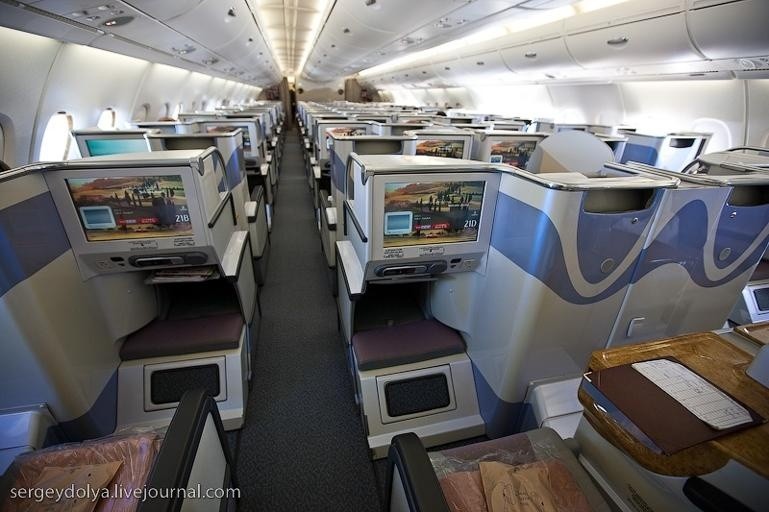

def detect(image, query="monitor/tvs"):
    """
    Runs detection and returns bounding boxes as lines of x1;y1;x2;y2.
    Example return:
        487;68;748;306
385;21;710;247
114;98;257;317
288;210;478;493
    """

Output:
64;175;194;242
206;125;251;152
490;141;536;170
416;139;464;159
86;138;148;156
325;127;366;151
384;181;486;248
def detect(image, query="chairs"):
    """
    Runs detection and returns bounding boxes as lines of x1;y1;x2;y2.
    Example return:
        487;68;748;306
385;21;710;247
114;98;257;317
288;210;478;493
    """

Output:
564;319;768;507
387;428;613;511
0;389;240;512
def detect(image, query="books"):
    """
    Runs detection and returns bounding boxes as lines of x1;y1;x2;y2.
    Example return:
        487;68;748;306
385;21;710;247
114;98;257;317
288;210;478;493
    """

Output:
581;355;768;460
